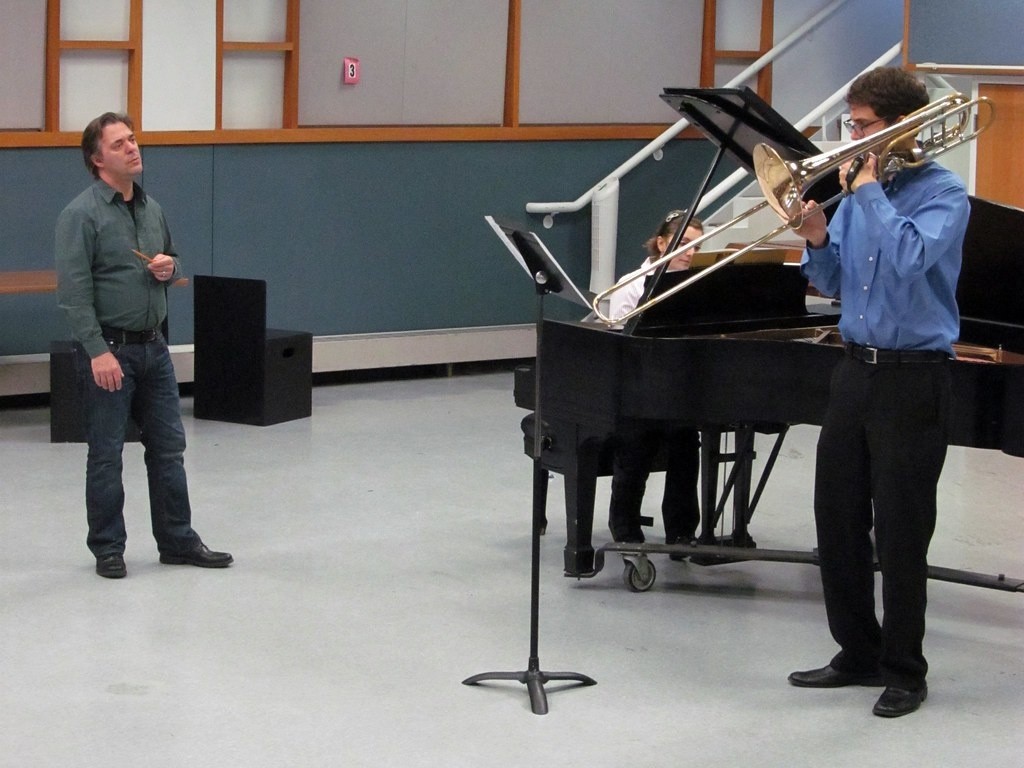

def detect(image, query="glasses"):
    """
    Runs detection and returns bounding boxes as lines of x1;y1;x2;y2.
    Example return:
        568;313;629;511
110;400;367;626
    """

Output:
844;115;889;138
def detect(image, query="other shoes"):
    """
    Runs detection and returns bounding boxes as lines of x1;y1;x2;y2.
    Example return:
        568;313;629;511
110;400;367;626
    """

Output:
607;519;646;544
663;534;699;548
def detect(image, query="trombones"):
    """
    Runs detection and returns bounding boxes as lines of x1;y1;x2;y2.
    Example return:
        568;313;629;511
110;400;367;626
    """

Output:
592;92;997;326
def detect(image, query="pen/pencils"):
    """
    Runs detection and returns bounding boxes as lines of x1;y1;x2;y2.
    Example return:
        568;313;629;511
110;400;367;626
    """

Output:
130;248;154;263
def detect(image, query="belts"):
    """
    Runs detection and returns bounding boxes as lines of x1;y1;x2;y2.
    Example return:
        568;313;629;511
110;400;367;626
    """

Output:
845;340;951;363
100;323;162;346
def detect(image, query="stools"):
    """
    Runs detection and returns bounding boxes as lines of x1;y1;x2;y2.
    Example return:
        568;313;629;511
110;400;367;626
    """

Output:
520;412;670;535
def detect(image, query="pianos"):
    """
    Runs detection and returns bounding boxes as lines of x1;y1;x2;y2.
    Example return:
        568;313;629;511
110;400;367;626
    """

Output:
512;85;1024;577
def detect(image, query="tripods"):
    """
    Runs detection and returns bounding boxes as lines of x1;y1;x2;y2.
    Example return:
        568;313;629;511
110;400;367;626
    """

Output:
461;226;597;716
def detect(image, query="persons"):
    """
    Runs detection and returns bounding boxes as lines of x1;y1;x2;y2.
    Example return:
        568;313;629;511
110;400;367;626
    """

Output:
608;209;704;561
55;111;234;579
787;67;973;715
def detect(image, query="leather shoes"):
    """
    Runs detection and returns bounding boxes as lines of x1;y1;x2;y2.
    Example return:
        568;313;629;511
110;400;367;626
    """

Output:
789;664;891;687
95;553;127;578
873;679;928;716
160;542;233;567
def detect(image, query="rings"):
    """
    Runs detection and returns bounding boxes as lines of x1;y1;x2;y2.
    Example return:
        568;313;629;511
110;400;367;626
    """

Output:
162;271;165;277
